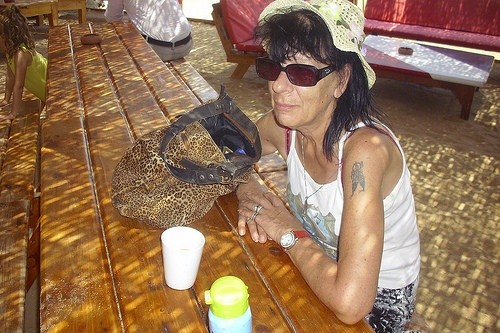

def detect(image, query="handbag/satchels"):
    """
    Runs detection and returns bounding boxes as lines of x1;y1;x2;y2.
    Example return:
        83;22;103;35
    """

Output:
111;84;262;228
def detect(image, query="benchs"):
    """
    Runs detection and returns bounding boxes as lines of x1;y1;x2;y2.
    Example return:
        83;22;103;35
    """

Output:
163;57;289;172
211;0;275;79
0;34;48;333
364;0;500;52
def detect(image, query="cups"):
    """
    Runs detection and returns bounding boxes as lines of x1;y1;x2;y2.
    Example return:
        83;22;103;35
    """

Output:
160;226;205;291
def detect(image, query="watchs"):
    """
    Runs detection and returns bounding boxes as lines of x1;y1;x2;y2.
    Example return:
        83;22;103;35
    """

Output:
280;231;311;251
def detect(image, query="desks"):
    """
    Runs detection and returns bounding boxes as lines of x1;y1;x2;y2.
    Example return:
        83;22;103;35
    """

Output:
360;33;495;120
15;0;86;26
39;19;377;333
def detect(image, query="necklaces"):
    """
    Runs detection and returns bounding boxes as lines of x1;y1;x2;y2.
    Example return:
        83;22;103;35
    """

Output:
301;134;337;214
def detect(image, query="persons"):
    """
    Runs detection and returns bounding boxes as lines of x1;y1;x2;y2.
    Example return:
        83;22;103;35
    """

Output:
105;0;193;61
235;0;420;333
0;4;48;121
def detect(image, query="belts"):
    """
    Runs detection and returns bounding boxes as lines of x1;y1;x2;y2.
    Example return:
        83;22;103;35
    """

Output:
142;33;191;47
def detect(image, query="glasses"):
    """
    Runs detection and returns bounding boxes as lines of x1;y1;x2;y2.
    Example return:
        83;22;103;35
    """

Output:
256;56;338;86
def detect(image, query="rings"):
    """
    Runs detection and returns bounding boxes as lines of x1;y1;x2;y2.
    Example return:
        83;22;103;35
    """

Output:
255;205;263;213
246;218;253;224
251;212;258;221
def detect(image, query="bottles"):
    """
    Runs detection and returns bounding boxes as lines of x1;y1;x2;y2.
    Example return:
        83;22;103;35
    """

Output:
203;276;254;333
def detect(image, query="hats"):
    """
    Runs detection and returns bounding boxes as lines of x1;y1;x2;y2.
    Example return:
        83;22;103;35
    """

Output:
258;0;376;90
